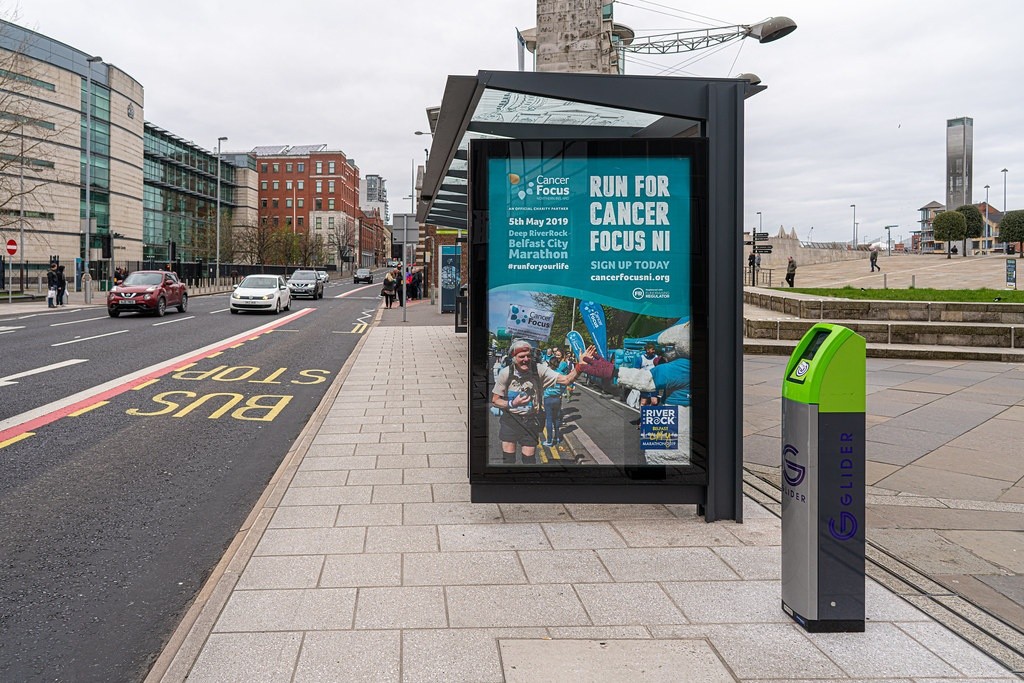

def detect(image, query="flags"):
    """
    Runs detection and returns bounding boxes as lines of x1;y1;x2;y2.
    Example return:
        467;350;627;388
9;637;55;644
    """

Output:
567;331;586;356
578;301;606;360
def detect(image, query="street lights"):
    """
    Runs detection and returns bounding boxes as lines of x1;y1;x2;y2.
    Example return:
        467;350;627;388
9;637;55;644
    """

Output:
885;227;891;256
1001;168;1008;253
984;185;990;248
17;119;29;263
217;137;228;292
864;236;867;244
855;223;859;250
84;56;102;304
850;205;855;249
757;212;762;232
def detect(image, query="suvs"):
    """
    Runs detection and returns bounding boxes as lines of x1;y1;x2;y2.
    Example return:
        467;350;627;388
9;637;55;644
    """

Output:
286;268;323;300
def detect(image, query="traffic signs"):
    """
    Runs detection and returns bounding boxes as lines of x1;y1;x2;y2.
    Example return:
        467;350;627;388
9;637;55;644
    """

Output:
755;233;769;241
744;241;755;245
755;245;773;253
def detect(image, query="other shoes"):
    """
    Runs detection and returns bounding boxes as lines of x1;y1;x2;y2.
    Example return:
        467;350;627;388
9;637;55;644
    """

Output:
389;307;392;309
49;304;57;308
384;307;388;309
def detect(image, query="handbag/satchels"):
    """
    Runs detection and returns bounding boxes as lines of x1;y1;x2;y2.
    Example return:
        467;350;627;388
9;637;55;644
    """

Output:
62;289;69;296
381;288;386;296
48;288;56;298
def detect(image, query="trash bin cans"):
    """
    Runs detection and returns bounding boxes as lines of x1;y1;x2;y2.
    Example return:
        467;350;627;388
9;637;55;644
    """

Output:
1007;244;1015;255
100;280;112;291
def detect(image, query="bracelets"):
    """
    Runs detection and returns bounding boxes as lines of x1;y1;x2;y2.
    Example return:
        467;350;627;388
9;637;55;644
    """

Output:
508;400;513;408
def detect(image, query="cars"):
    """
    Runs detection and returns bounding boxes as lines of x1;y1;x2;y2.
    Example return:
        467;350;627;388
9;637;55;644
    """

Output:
354;268;373;284
317;271;329;283
107;270;188;317
388;262;398;268
229;274;292;315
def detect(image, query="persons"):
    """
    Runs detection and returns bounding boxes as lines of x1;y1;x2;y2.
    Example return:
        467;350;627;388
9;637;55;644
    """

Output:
756;253;761;267
115;267;129;285
490;322;690;465
383;262;422;309
870;248;880;272
47;263;66;307
951;245;958;253
786;256;797;287
747;251;754;273
231;267;238;284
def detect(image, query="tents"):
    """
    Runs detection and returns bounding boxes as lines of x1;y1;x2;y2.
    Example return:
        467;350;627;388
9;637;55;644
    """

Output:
624;315;689;349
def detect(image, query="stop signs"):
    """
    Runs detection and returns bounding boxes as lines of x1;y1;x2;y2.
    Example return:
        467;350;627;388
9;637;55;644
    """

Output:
6;240;18;255
